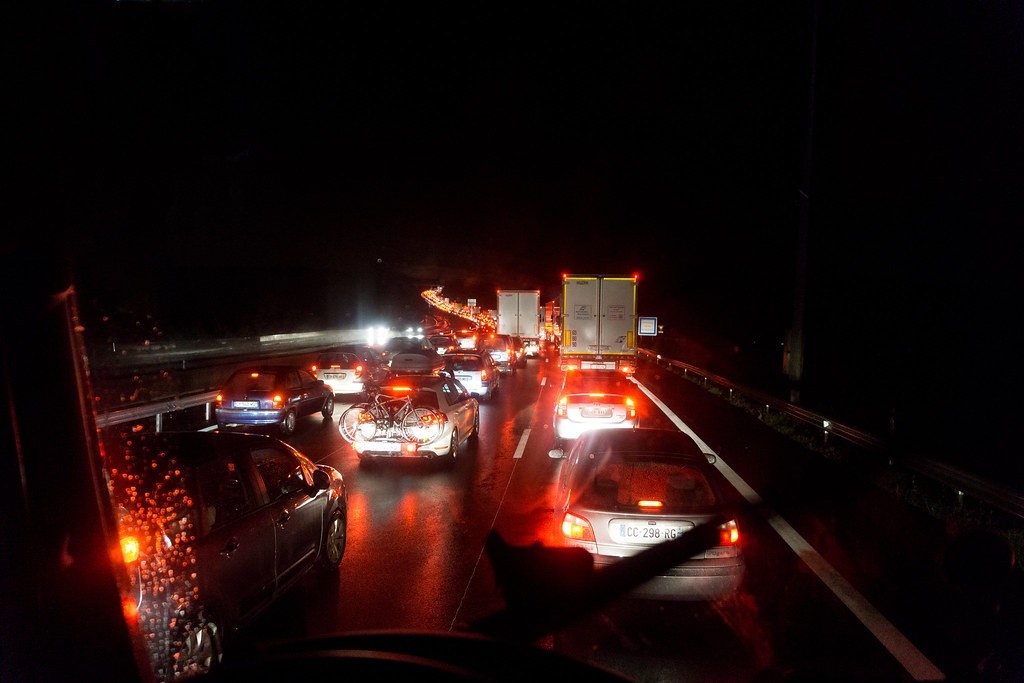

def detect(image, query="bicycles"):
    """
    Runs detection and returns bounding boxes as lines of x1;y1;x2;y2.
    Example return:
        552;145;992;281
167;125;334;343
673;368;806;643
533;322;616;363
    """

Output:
338;372;444;446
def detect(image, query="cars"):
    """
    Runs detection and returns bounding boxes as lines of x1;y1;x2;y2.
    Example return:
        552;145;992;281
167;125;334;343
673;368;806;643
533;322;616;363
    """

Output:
511;336;527;367
523;340;539;359
351;346;388;378
430;334;461;353
474;334;516;376
422;286;497;328
391;347;445;373
97;431;348;679
215;366;336;436
314;351;373;401
548;429;747;603
552;369;641;447
440;349;500;400
384;335;435;364
353;371;480;463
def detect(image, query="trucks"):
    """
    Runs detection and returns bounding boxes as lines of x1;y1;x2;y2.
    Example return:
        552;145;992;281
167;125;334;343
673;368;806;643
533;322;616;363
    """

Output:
496;288;540;339
559;274;638;376
540;301;560;349
